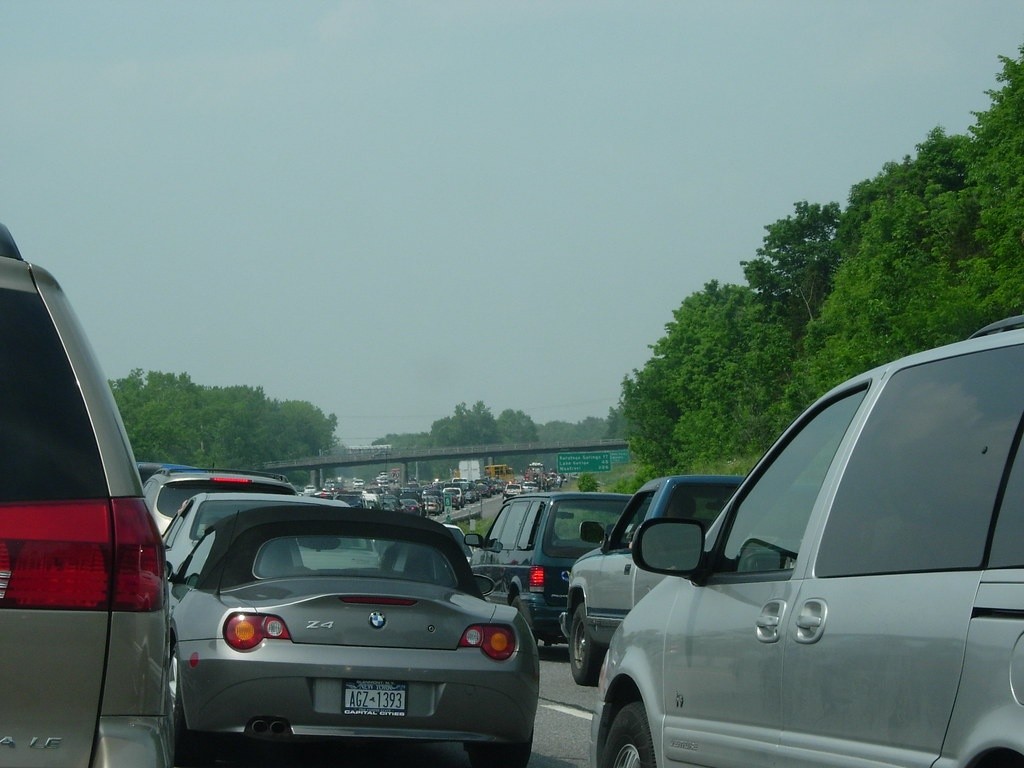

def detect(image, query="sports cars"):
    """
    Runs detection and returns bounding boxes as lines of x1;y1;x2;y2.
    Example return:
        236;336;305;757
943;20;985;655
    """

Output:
163;502;539;768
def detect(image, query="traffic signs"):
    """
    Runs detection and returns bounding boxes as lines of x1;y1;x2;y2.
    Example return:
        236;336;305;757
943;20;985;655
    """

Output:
605;449;630;464
557;452;611;475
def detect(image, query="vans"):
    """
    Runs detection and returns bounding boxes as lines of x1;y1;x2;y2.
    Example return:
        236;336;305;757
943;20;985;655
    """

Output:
464;488;633;659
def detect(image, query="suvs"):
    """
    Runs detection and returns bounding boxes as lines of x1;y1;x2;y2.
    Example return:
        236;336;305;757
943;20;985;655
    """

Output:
138;467;300;561
559;474;757;687
585;317;1024;766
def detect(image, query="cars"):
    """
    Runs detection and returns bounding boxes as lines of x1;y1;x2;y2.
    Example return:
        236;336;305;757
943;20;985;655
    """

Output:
131;461;195;498
1;220;183;766
301;451;565;517
440;523;477;570
163;493;357;620
